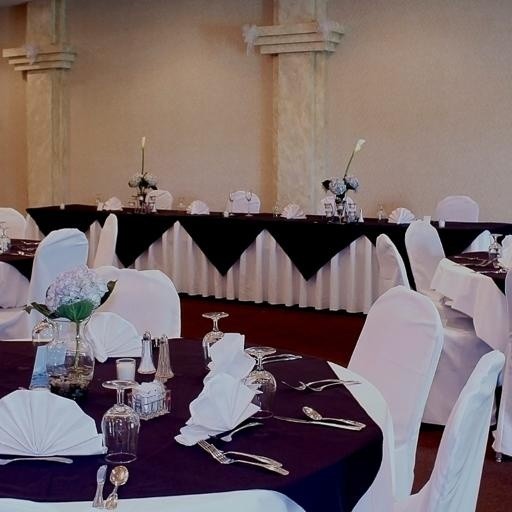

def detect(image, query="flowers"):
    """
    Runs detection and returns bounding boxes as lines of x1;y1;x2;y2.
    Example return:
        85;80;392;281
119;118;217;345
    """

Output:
127;135;159;191
23;263;120;324
318;136;370;199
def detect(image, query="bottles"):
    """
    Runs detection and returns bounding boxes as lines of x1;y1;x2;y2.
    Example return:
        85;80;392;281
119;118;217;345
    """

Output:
155;335;174;379
137;329;157;374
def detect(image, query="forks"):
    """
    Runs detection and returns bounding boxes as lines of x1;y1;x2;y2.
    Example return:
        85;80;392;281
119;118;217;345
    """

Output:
460;260;492;267
206;444;290;476
281;379;342;391
208;422;263;443
197;439;283;467
299;380;361;392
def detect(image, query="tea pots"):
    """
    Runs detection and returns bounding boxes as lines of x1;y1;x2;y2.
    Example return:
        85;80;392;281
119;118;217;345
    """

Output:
30;315;95;401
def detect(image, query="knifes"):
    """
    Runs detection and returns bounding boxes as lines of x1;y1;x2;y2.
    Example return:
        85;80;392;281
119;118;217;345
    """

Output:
256;352;301;366
91;464;109;507
274;415;362;431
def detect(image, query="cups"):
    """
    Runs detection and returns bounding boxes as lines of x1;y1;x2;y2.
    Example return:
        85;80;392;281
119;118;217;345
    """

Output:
116;358;137;395
438;216;446;228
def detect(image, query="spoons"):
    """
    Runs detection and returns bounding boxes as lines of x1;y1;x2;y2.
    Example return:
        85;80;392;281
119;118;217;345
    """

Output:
303;406;367;428
0;456;74;466
106;465;128;510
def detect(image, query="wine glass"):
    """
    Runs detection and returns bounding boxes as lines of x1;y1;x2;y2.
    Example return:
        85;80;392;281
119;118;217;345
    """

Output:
489;233;502;259
101;380;141;464
201;312;228;369
60;189;384;222
245;346;276;419
0;221;12;254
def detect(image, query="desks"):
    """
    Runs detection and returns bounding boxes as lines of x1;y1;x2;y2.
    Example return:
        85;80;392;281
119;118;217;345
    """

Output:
28;201;511;318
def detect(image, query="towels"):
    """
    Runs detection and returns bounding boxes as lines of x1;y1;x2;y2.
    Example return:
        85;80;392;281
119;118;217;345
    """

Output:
1;386;114;458
386;206;414;226
85;309;145;365
280;202;307;221
172;368;268;446
207;331;255;377
186;198;209;216
101;197;123;211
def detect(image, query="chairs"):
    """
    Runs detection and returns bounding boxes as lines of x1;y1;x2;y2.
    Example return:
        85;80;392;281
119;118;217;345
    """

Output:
145;190;176;212
389;348;506;510
427;193;482;223
225;190;261;214
375;220;510;465
345;284;447;495
68;266;185;343
0;206;128;309
0;227;91;341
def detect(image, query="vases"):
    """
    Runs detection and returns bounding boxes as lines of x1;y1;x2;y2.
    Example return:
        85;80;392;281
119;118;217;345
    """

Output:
135;193;148;213
43;319;95;394
334;201;346;222
